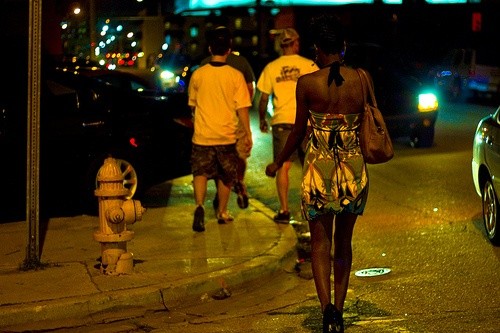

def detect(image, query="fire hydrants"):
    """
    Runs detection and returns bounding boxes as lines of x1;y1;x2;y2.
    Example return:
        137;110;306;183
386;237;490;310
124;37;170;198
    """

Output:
94;158;147;276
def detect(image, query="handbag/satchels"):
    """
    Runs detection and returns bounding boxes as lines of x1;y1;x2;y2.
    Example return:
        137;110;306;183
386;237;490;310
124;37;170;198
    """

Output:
355;68;394;164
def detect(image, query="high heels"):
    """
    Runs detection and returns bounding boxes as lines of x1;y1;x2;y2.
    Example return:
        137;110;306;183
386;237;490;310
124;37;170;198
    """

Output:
337;312;344;332
323;303;339;332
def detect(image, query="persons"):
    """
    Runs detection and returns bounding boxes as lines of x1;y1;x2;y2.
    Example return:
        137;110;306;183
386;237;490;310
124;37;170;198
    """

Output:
187;31;252;229
257;28;320;224
200;24;255;213
264;20;377;332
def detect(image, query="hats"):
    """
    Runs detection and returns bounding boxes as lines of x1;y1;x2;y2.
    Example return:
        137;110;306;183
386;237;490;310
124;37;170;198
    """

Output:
279;28;300;45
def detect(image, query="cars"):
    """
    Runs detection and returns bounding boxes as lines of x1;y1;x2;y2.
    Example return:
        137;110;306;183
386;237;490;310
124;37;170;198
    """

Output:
365;78;438;148
171;54;190;76
1;66;192;205
472;107;500;245
231;50;263;71
429;49;500;102
49;58;153;86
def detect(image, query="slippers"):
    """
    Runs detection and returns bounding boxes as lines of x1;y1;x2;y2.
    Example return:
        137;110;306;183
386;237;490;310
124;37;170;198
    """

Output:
192;204;205;232
216;211;234;224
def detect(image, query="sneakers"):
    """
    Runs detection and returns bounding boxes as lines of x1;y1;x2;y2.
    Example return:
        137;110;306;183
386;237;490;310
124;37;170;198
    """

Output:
274;209;290;223
233;180;249;209
